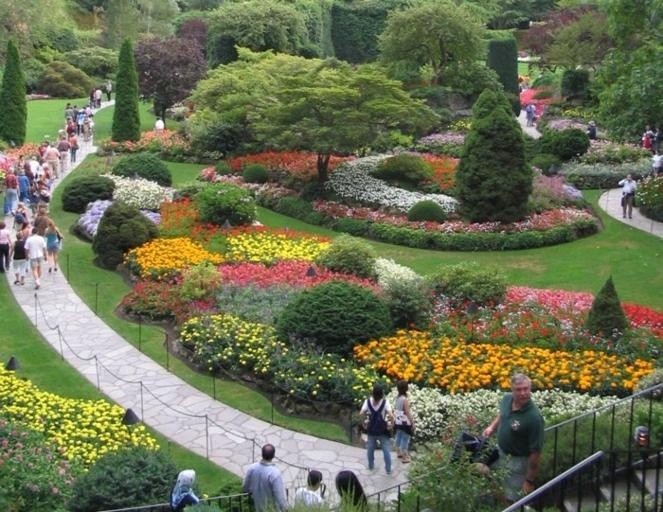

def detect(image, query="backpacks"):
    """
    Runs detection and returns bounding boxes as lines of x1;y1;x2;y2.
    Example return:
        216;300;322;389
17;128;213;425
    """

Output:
367;398;386;435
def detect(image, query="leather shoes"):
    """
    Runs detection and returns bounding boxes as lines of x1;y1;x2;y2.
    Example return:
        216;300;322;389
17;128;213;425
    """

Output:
13;267;58;289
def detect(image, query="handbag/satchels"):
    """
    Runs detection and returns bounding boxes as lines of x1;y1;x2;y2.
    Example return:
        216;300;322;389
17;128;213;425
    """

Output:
393;409;411;426
73;144;79;149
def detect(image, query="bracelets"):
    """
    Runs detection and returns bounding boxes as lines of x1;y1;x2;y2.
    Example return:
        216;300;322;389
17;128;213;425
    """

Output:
525;478;535;485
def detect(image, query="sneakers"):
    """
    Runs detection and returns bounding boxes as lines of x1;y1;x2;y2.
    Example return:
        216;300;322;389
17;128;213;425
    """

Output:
397;455;411;463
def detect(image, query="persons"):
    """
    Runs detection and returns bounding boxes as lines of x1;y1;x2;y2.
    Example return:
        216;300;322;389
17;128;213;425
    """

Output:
0;87;101;291
105;80;112;102
525;103;533;127
392;378;416;463
357;383;395;475
480;372;545;502
154;117;166;135
642;124;662;177
528;102;537;127
170;468;200;511
289;469;325;506
241;443;291;512
617;174;637;219
586;120;596;139
538;71;545;78
523;74;529;89
331;470;370;511
